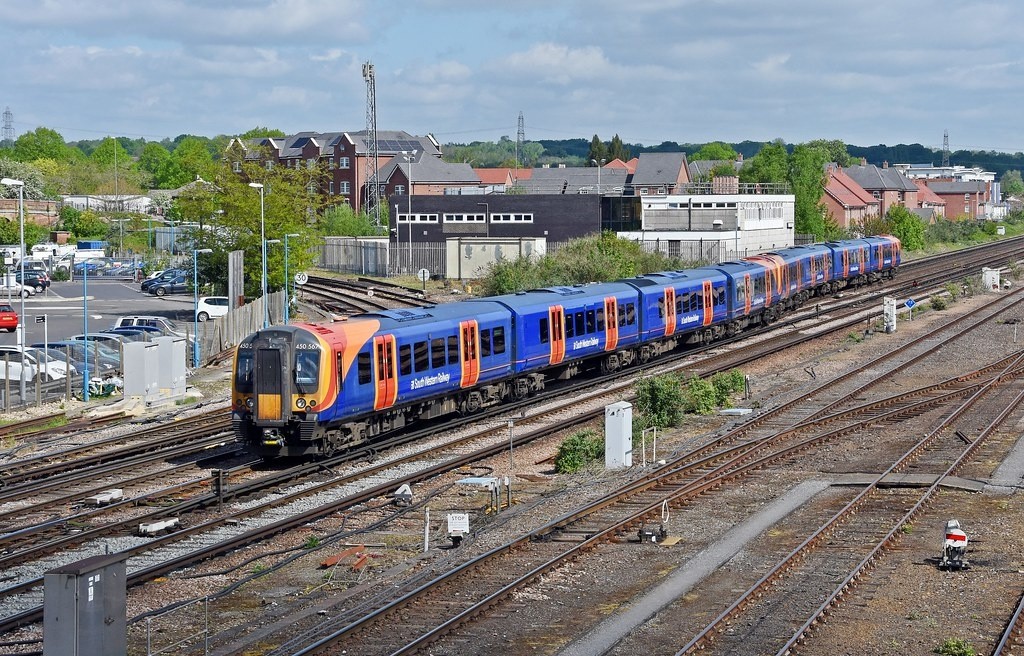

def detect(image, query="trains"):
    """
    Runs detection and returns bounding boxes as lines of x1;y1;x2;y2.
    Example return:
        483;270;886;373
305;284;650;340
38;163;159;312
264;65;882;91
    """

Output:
229;233;903;462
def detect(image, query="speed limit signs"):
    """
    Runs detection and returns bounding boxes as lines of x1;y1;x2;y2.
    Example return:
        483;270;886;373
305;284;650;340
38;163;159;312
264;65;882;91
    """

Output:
293;272;308;285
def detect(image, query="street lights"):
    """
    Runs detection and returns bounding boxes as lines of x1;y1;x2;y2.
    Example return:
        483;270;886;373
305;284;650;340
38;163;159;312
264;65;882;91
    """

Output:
477;202;489;237
262;240;281;328
0;176;27;408
400;149;419;276
283;234;300;325
248;181;266;295
111;136;118;212
73;261;90;402
590;159;607;195
193;248;213;369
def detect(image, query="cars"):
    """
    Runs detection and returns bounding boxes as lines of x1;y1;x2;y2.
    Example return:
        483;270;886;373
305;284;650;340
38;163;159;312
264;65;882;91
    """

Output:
197;296;230;322
0;315;200;395
12;248;210;296
0;303;19;332
0;277;37;299
